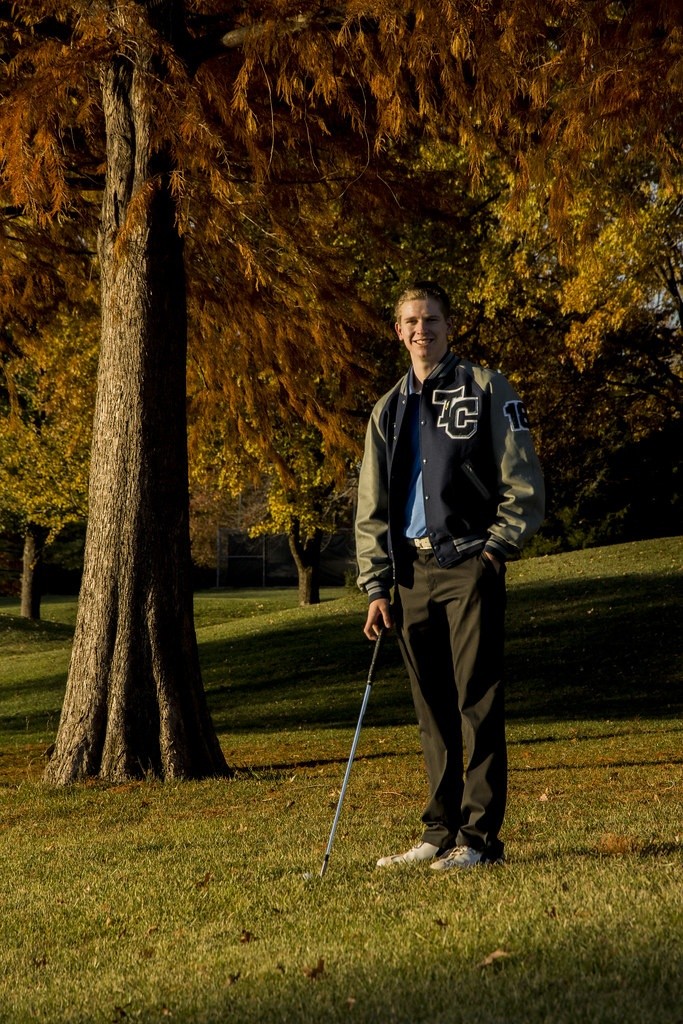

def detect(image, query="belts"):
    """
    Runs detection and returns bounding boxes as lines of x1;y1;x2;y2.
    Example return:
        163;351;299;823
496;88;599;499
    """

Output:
406;536;432;550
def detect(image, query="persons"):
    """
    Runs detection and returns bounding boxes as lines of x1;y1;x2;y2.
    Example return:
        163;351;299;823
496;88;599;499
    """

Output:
356;279;543;872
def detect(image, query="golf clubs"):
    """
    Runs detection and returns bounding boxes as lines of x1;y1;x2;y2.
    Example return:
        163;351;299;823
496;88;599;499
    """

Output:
301;624;387;885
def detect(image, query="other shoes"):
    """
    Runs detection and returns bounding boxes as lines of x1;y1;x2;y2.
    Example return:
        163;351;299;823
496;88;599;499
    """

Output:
429;845;506;870
376;840;457;869
